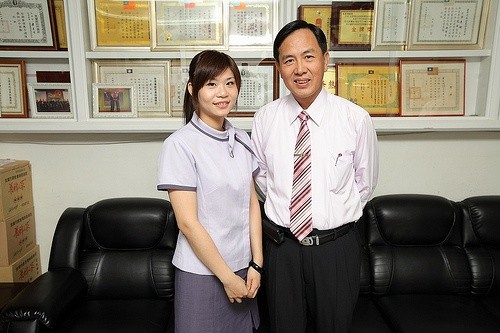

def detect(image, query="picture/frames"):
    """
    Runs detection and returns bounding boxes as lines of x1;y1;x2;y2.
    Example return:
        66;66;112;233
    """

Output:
26;83;73;119
92;83;137;118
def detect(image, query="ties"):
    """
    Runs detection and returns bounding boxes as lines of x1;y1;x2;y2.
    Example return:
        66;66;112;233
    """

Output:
290;111;313;241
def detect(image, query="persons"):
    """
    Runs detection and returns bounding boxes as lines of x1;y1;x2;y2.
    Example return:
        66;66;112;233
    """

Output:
35;98;70;112
252;19;379;333
152;47;264;333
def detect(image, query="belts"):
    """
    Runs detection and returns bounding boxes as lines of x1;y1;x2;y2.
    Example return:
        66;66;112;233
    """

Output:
283;222;355;246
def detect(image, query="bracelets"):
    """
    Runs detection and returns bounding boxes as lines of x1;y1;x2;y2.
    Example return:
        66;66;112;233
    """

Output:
249;261;263;274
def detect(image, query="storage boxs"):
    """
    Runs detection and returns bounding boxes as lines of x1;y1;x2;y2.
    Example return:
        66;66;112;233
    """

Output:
0;159;41;283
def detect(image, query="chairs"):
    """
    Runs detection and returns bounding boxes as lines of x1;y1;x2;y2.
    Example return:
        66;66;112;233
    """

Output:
4;198;177;333
356;193;500;333
